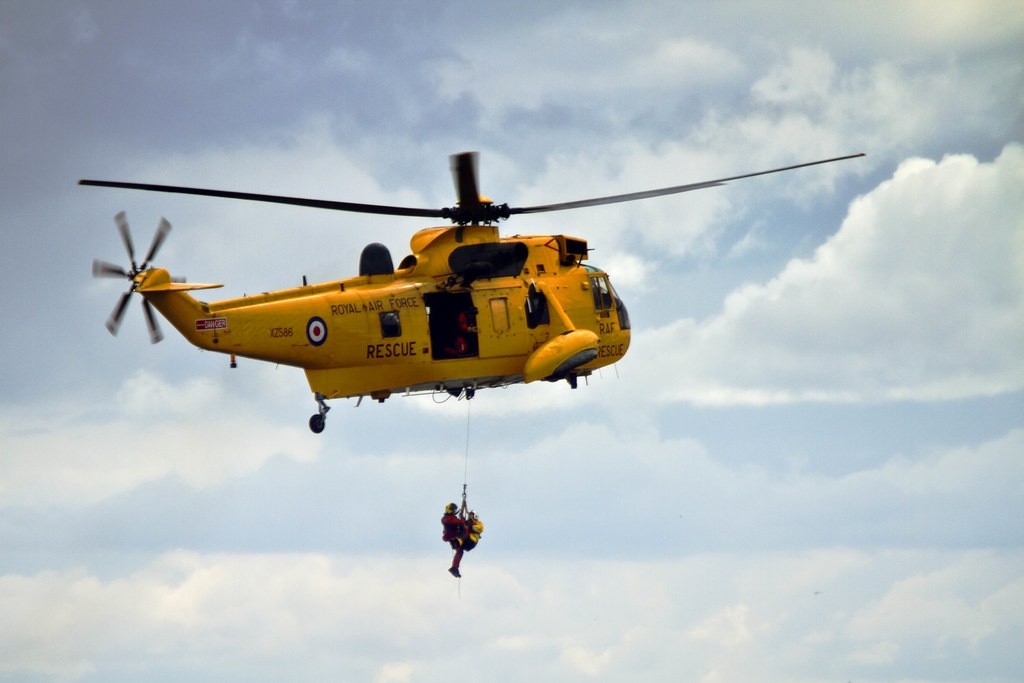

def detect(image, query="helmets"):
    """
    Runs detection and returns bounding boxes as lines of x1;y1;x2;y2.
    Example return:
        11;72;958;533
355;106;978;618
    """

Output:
444;503;458;514
468;510;479;520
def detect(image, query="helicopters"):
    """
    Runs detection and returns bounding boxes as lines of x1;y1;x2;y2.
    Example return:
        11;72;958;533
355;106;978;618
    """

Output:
76;148;865;435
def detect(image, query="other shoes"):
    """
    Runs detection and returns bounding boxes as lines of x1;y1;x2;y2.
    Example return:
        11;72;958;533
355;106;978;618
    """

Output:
448;567;461;578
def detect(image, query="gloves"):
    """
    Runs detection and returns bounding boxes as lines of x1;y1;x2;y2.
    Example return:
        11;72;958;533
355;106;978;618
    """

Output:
472;326;480;333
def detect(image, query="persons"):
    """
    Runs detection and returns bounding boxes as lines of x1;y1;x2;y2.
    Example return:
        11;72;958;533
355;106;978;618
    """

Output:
441;503;484;577
443;304;478;354
